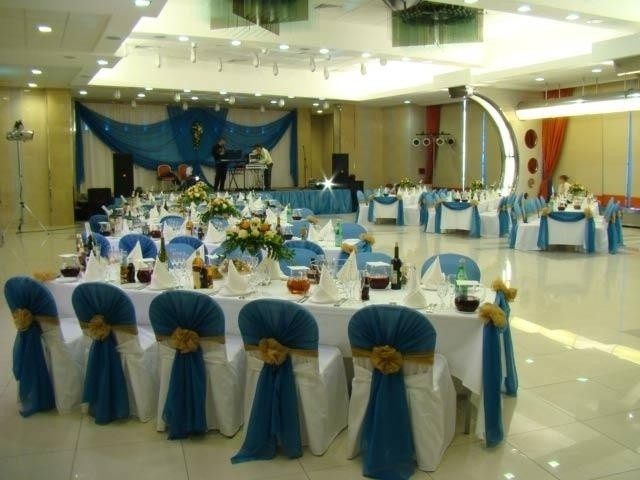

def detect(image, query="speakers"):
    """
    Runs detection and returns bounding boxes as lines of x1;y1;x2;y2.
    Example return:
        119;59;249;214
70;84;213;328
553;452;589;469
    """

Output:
113;152;134;198
88;187;111;201
332;153;348;182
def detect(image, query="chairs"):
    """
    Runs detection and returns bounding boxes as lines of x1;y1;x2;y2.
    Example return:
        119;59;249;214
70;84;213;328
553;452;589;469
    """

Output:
177;163;189;185
279;242;327;276
146;289;243;437
211;242;264;266
419;253;483;286
83;187;624;251
76;231;114;262
232;299;350;459
156;164;176;191
4;274;84;418
118;233;158;262
160;243;197;268
337;252;398;283
69;284;158;427
343;308;461;477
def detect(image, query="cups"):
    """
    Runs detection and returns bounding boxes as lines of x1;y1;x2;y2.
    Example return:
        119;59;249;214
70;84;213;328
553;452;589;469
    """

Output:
455;286;481;312
61;257;80;276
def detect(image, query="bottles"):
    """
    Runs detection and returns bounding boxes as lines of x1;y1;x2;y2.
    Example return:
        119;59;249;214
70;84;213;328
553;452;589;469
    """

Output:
121;210;467;300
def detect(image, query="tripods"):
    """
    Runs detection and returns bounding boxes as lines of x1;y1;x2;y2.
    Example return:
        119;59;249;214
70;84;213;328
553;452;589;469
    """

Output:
0;143;51;239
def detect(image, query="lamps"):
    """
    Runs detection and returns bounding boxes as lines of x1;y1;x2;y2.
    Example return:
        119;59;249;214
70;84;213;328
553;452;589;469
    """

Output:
516;76;640;121
412;131;455;148
114;42;401;115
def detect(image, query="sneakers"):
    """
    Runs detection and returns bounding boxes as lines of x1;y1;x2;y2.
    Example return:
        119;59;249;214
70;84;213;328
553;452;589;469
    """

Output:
220;189;227;191
214;189;218;193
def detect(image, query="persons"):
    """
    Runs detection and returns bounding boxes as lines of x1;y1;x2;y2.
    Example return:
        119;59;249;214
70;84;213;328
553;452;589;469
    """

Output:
251;143;274;190
557;174;572;197
211;137;228;193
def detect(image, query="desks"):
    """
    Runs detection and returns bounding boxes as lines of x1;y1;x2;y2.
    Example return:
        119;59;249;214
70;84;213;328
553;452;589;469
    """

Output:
38;265;520;443
